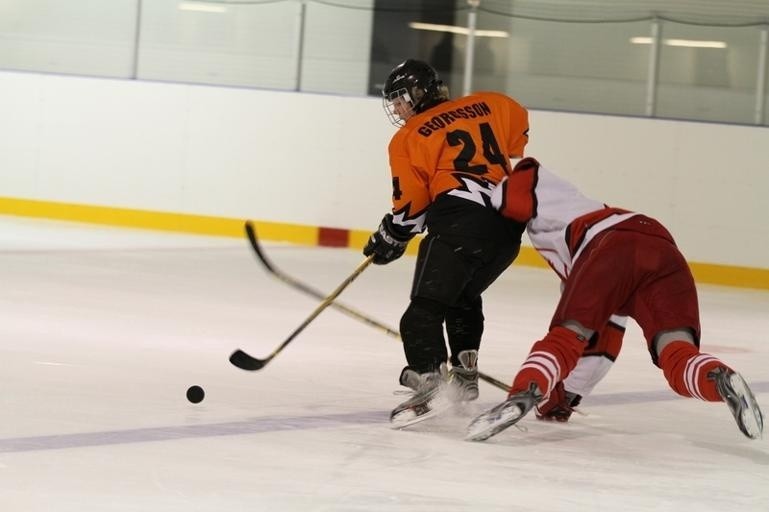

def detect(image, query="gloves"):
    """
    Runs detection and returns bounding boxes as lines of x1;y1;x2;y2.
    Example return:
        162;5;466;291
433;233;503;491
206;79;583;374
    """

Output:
363;212;416;265
534;389;583;423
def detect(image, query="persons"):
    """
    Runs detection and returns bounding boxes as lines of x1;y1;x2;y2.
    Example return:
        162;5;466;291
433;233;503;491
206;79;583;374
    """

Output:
468;159;764;443
364;58;529;428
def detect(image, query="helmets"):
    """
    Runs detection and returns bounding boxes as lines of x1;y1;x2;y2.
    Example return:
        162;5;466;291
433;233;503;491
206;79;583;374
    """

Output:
382;56;449;114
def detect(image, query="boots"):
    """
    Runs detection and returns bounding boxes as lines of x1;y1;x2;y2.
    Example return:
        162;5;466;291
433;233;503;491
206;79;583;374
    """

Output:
391;349;479;424
467;382;541;442
708;367;764;439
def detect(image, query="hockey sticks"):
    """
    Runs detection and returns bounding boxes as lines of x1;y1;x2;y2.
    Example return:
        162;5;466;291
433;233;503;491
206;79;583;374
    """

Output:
229;245;374;372
244;220;513;393
